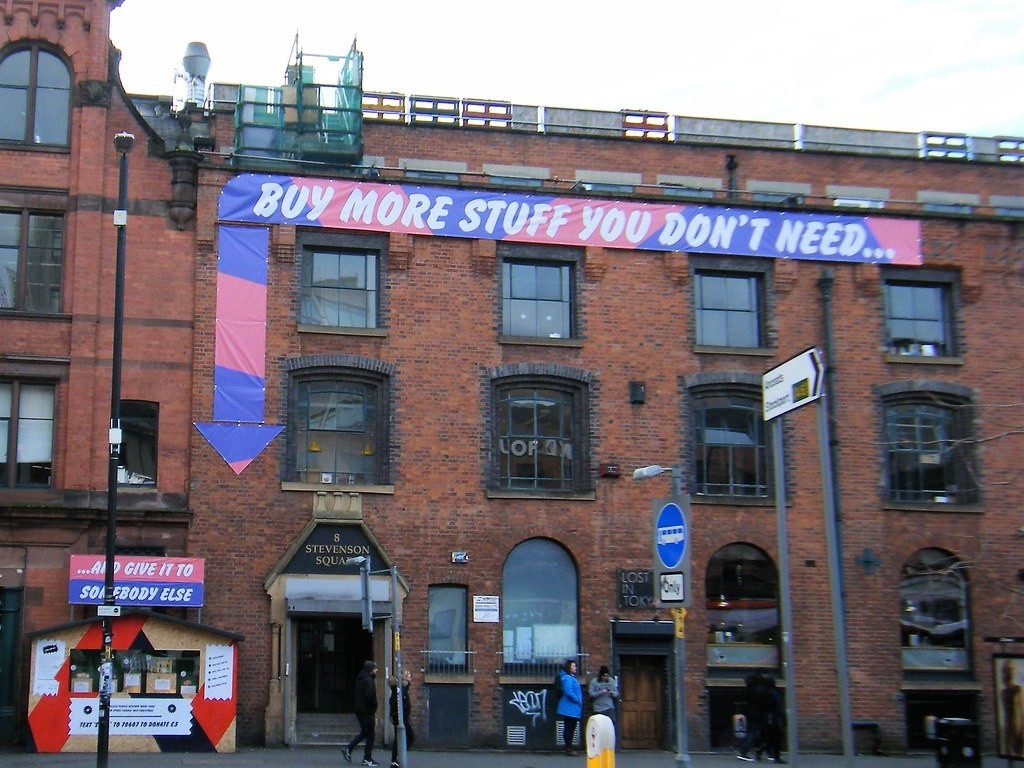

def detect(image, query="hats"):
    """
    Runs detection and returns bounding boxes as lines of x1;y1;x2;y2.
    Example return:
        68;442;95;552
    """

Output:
363;660;377;672
599;664;609;677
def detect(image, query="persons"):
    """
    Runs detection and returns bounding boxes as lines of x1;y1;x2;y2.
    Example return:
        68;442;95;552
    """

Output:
589;666;620;732
556;660;583;756
341;660;379;767
388;669;415;768
736;667;788;764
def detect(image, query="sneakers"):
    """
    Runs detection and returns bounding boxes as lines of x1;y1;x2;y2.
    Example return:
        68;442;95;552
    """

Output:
361;757;379;767
341;746;353;763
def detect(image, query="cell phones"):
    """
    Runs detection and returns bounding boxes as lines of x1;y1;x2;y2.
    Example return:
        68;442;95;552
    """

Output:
607;690;610;691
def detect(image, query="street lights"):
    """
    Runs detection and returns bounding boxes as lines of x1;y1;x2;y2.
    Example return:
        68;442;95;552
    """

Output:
96;131;136;767
632;463;692;768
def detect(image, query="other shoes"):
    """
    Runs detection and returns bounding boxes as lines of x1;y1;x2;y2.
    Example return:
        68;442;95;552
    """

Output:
390;761;400;768
735;754;754;762
565;749;580;757
774;757;788;765
766;755;785;761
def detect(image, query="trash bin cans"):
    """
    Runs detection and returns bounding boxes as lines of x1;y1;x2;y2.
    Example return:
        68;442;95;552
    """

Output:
934;717;981;768
851;721;880;755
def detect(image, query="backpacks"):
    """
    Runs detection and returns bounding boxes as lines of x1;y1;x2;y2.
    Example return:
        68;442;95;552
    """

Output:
555;675;566;697
